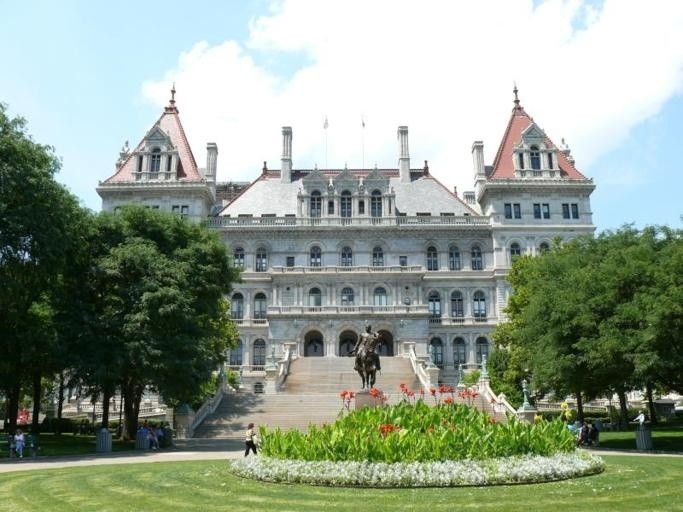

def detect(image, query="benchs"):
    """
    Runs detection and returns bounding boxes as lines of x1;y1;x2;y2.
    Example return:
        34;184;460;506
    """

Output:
8;435;39;460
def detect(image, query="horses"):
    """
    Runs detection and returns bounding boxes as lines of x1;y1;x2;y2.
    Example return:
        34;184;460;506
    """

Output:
357;335;378;387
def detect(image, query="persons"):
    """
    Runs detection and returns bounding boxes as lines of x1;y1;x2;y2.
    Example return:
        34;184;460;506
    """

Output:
245;423;258;457
352;323;381;369
633;410;646;425
567;420;599;449
143;420;170;450
79;419;91;436
14;429;26;458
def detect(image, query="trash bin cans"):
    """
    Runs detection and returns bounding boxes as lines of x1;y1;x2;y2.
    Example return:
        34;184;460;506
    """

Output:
135;429;149;449
636;425;651;449
96;428;111;453
